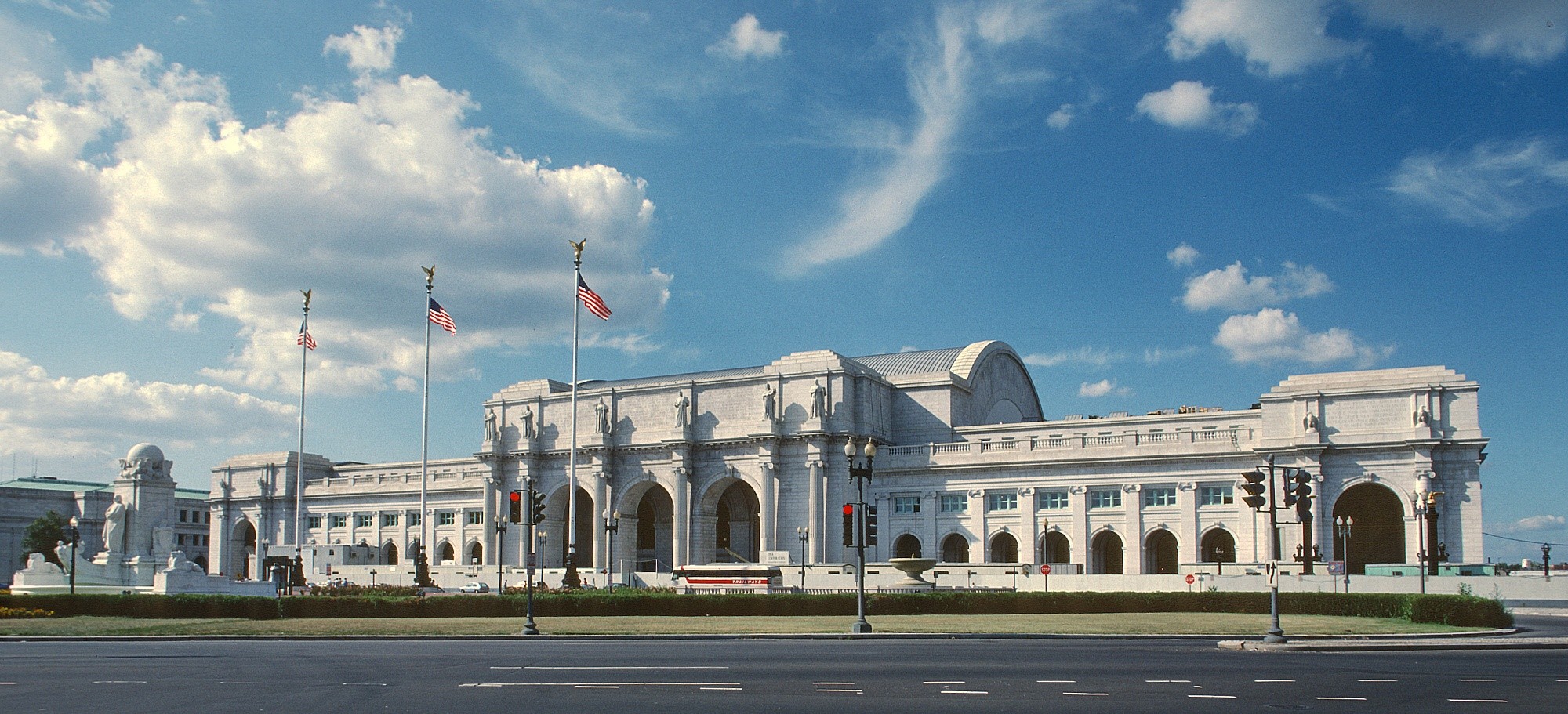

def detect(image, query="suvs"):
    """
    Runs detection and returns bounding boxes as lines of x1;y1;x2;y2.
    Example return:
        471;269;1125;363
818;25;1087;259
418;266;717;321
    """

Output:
459;581;548;594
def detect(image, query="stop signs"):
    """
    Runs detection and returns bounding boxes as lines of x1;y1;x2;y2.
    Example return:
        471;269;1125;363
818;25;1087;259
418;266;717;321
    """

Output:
1041;564;1050;574
1185;574;1194;584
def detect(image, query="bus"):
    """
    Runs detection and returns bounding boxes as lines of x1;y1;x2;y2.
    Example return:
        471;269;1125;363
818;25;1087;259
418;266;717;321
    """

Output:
670;562;783;597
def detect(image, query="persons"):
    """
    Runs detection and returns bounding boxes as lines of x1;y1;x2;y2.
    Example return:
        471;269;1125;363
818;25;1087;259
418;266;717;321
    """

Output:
326;577;348;590
519;406;534;439
101;494;126;552
593;396;609;433
674;391;689;427
809;379;827;418
483;408;497;441
53;540;85;574
762;383;777;420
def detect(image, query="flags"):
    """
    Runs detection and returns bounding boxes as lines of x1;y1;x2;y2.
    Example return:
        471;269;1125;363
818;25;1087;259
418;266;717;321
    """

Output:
429;297;456;337
297;319;317;350
578;272;612;320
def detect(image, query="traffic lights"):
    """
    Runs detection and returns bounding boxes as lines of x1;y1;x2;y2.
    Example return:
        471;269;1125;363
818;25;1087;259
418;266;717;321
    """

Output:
510;492;546;522
1241;468;1311;511
843;504;878;546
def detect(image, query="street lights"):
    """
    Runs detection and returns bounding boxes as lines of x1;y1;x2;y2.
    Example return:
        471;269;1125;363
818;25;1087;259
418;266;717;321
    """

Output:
1409;488;1430;593
602;509;621;587
262;538;270;580
1541;543;1551;576
844;436;875;633
70;516;79;595
494;514;547;594
1336;515;1353;593
1215;546;1224;577
797;526;809;588
1043;518;1048;592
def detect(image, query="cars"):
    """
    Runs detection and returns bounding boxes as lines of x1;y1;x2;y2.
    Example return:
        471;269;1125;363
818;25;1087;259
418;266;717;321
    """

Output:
580;584;597;590
305;582;357;591
603;583;626;589
